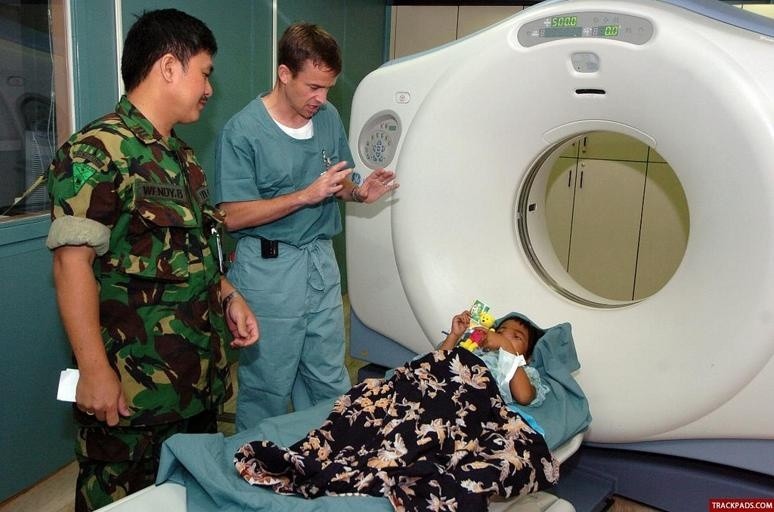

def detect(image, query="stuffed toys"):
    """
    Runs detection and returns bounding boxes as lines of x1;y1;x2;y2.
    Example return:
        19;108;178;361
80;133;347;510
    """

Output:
459;312;495;352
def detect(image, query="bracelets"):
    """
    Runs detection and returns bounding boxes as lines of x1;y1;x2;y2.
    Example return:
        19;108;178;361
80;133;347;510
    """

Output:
350;185;360;202
223;291;239;309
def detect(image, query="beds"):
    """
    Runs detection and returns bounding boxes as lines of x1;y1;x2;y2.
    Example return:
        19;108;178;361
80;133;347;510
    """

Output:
89;355;618;510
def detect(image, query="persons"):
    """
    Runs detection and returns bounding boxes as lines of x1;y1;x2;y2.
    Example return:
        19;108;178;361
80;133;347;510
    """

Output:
46;8;259;512
440;310;550;408
213;21;400;435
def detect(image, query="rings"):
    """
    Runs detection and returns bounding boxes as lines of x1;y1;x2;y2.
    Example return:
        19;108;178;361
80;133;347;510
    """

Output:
86;411;95;416
